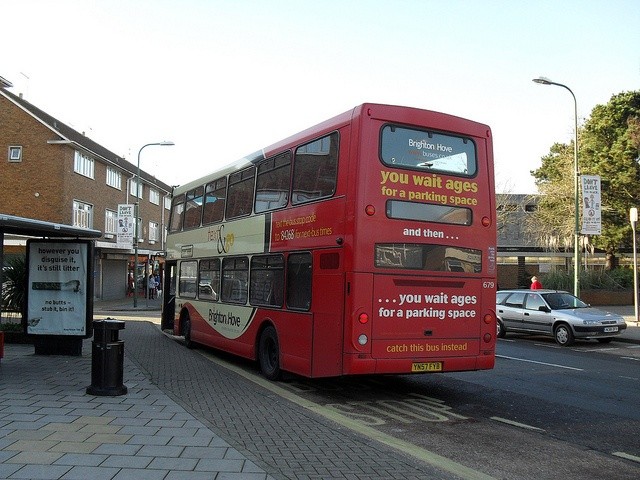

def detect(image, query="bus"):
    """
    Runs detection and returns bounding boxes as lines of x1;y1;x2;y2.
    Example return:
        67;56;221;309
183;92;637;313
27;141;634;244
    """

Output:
160;102;496;380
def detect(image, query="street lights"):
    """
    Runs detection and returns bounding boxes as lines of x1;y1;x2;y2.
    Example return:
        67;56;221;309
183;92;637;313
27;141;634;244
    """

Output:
532;77;581;307
134;142;175;309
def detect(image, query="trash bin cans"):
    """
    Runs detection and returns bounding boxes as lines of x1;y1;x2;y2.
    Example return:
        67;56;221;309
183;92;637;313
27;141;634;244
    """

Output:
86;319;127;396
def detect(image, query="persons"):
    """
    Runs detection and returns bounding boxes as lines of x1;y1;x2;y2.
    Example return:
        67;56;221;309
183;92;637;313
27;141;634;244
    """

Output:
148;274;156;299
530;276;542;289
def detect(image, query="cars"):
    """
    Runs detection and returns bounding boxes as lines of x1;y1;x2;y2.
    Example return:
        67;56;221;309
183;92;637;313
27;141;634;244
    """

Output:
495;289;627;347
